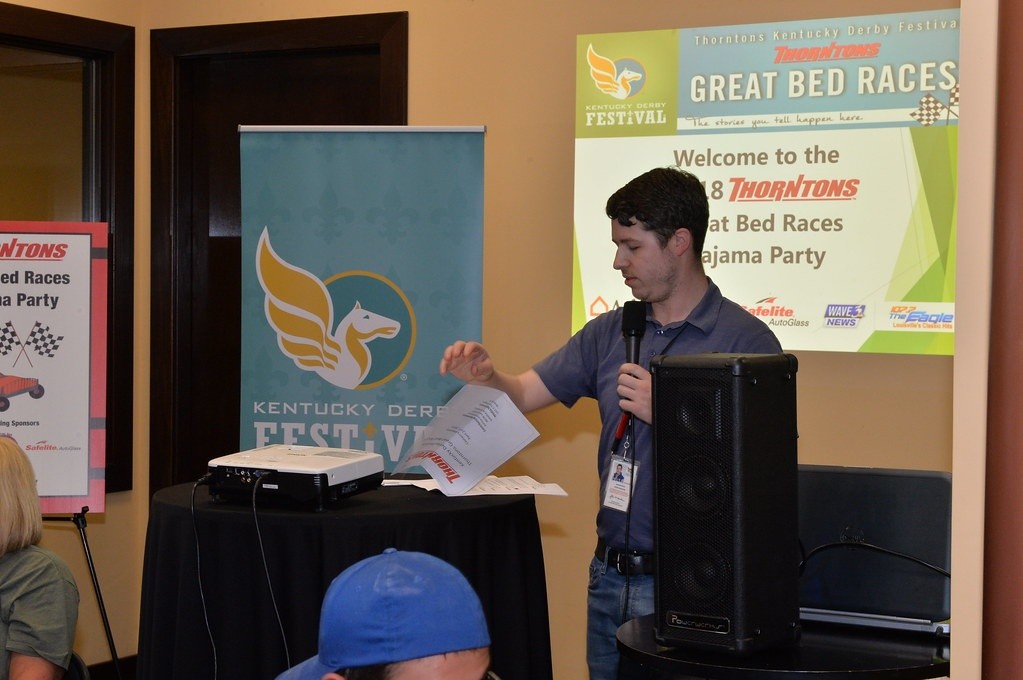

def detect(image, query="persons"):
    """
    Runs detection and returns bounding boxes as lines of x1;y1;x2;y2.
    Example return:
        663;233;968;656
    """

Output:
440;167;784;680
613;464;624;482
273;547;492;680
0;435;80;680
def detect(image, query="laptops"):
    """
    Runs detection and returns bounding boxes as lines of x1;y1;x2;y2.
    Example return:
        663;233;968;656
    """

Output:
796;461;953;637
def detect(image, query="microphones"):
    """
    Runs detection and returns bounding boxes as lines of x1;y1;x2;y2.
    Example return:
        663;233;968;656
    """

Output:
622;300;647;416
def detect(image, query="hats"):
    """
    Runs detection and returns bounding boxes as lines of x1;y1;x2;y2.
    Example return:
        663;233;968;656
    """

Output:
275;548;491;680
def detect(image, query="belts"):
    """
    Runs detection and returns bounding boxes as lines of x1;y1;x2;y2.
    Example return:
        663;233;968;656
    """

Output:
594;537;653;576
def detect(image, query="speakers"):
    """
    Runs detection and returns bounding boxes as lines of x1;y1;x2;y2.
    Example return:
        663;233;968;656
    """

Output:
649;351;802;659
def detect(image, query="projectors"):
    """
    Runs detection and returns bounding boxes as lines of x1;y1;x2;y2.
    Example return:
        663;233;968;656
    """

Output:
208;444;385;515
